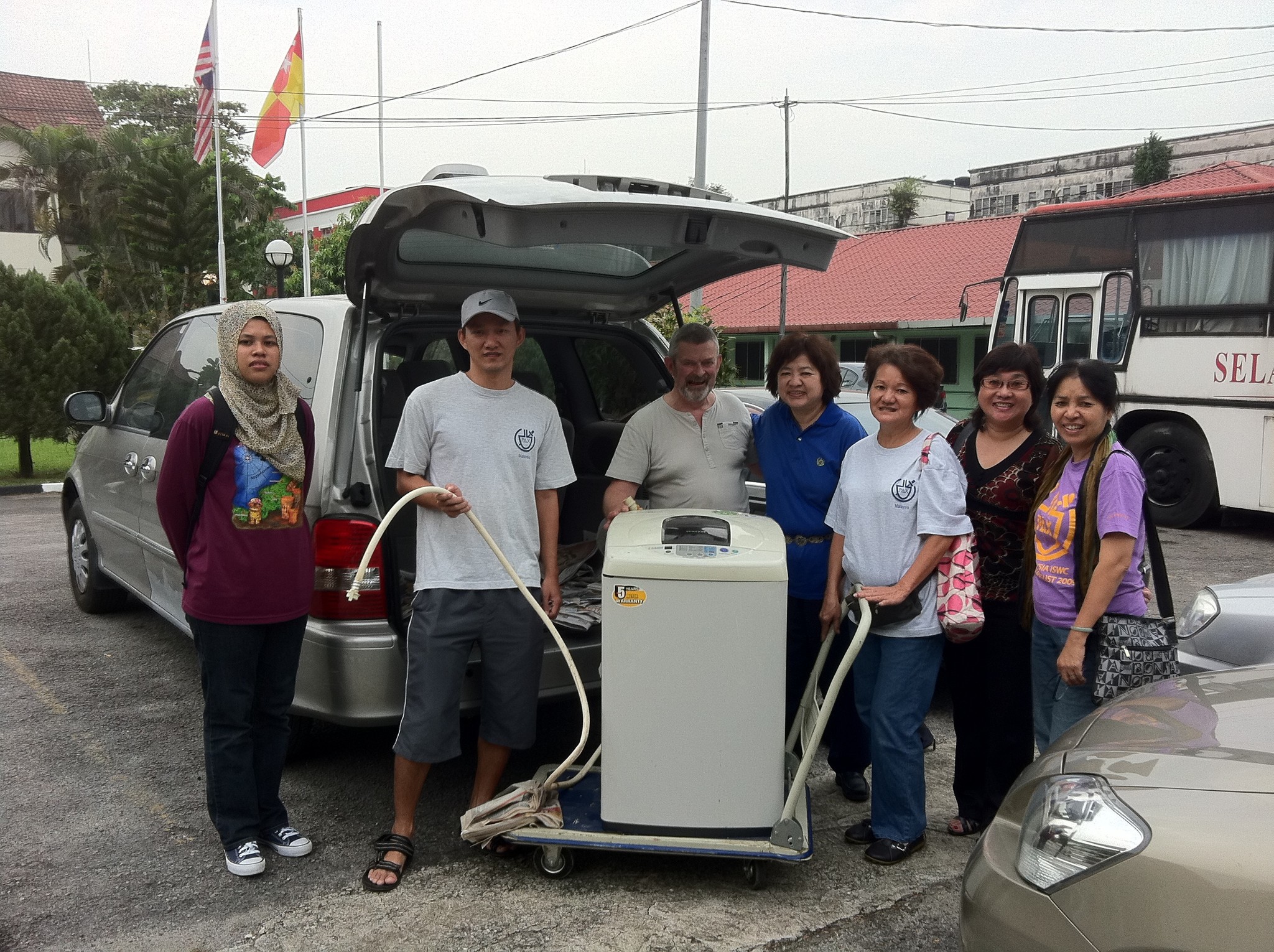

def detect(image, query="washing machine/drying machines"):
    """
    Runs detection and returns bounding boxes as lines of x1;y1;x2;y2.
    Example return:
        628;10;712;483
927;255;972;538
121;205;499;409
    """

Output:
592;502;786;833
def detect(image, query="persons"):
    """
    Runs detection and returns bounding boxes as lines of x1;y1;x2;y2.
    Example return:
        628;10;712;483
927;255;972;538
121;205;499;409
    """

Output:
155;302;316;878
602;322;766;529
1024;359;1148;753
819;342;974;865
361;287;577;892
749;331;873;803
946;342;1152;837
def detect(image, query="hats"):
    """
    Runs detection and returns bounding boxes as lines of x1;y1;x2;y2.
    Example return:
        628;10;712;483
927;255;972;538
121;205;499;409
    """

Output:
460;290;520;330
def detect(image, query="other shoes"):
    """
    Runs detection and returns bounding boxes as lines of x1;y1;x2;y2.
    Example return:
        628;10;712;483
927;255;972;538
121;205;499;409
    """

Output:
843;817;881;844
863;833;926;864
835;770;870;803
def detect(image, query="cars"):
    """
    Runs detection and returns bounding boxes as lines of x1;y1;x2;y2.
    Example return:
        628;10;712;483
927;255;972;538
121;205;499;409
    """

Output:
835;361;869;393
710;386;964;517
955;659;1274;951
1175;572;1274;679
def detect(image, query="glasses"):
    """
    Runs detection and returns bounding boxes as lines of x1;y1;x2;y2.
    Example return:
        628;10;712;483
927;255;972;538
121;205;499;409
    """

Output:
979;376;1033;391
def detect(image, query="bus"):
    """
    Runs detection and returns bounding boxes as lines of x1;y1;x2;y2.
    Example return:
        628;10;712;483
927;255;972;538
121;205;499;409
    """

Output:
956;179;1274;537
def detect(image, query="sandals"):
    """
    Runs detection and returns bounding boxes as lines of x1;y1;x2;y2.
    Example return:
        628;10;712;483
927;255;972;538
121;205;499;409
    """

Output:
946;814;980;836
361;833;413;891
460;835;521;858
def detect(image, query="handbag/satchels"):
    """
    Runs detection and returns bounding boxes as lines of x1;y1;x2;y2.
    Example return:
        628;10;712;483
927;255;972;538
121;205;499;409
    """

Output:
1091;611;1184;706
936;529;986;643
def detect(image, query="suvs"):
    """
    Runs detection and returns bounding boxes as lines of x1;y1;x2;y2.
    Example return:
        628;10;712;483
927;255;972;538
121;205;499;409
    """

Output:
57;167;864;749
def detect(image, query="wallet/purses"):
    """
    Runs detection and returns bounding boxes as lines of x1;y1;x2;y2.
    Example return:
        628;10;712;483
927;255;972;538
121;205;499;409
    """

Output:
847;581;922;628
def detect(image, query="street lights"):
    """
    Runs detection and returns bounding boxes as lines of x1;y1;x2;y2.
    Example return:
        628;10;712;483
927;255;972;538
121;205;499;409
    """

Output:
264;239;295;302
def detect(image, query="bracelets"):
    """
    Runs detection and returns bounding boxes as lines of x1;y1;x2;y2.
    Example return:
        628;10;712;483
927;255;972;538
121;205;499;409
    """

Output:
1070;625;1094;632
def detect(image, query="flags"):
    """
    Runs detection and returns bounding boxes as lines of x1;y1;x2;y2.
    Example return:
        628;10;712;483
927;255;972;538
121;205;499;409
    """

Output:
194;4;219;165
252;30;307;168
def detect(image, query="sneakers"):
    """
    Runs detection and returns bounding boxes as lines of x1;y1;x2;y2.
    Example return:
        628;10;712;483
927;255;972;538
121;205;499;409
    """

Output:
224;838;267;877
258;824;313;858
